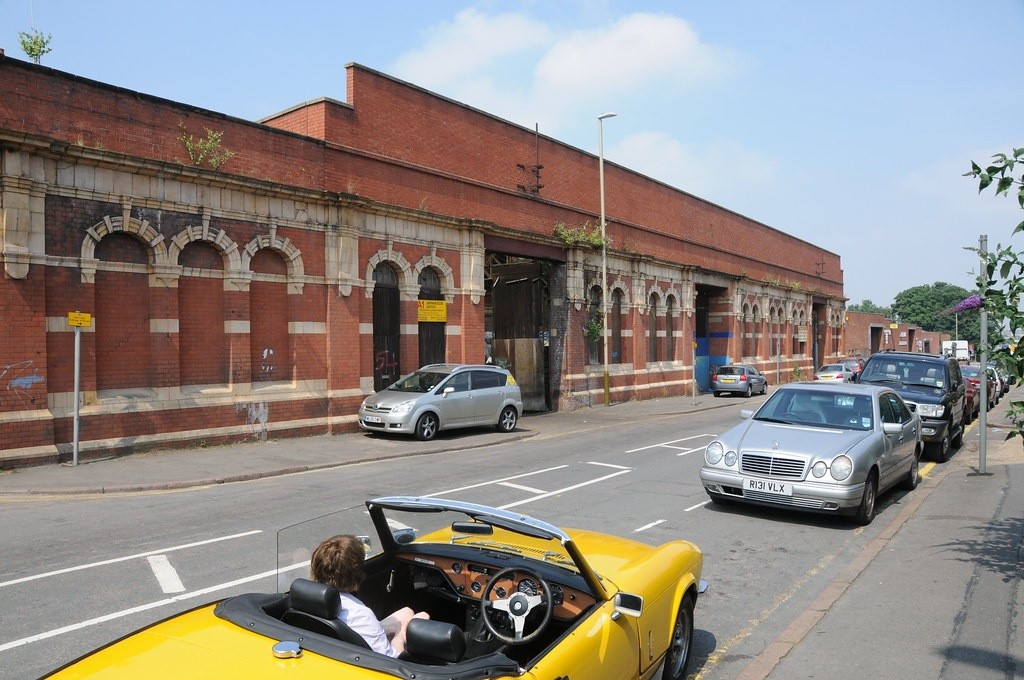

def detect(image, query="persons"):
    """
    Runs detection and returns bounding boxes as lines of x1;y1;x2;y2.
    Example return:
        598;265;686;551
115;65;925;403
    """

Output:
789;393;829;425
484;331;493;365
310;534;431;661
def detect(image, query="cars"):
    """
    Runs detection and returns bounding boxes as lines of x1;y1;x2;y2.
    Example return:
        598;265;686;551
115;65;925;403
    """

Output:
956;359;1013;425
837;356;869;381
701;379;924;527
815;364;861;378
713;365;768;399
358;363;523;441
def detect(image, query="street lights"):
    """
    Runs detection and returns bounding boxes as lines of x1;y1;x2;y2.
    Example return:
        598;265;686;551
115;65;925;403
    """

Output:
599;110;618;406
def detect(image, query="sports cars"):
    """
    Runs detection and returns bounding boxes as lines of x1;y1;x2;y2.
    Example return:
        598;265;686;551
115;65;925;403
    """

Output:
33;493;704;680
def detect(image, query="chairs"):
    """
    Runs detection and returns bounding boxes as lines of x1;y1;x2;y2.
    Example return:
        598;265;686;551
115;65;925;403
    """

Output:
405;619;466;666
845;397;872;427
920;368;942;385
454;375;465;391
280;580;372;650
880;364;900;379
787;392;827;423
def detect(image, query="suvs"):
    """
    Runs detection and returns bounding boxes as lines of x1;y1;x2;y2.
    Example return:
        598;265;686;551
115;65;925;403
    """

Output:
857;347;968;462
